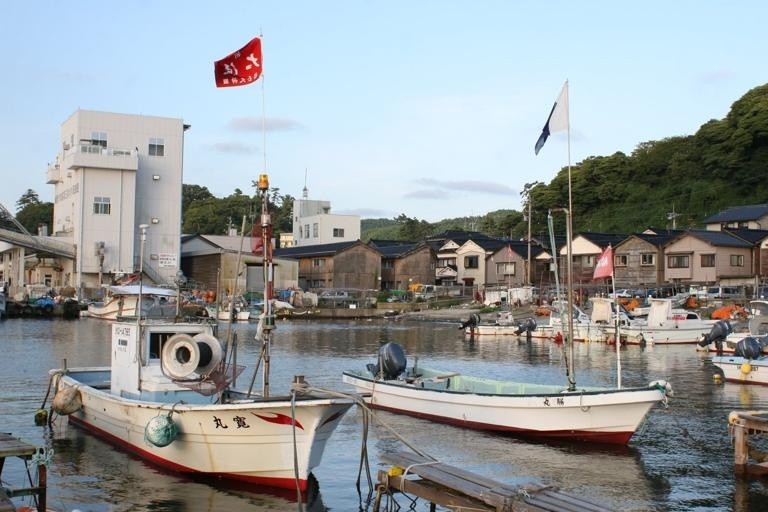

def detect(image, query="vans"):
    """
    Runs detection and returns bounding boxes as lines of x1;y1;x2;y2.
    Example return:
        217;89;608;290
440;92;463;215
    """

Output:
709;286;741;298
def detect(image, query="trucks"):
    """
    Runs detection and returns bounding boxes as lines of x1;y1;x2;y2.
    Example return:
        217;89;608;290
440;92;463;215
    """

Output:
415;285;461;301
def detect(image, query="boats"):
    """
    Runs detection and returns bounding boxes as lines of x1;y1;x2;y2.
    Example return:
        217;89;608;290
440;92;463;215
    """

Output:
458;299;739;344
87;294;155;318
697;295;768;385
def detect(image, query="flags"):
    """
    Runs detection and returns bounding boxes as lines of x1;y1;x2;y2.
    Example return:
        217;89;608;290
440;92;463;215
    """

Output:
592;246;614;280
533;81;570;156
213;35;262;87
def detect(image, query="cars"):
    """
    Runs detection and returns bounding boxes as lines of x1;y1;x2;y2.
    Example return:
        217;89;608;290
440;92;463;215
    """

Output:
610;286;679;298
317;289;377;306
